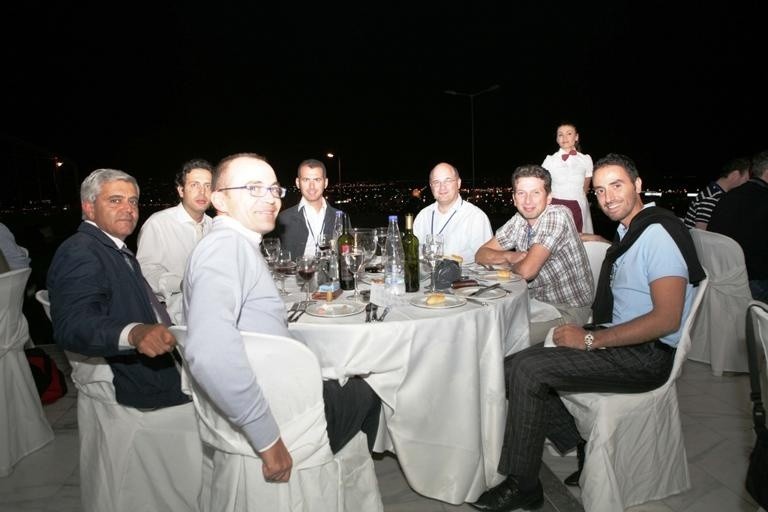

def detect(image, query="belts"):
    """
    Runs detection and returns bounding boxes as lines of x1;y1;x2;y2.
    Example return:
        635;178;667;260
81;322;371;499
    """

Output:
654;338;676;354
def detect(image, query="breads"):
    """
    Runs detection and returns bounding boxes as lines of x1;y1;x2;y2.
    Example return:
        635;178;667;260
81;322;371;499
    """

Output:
495;267;511;279
422;294;445;305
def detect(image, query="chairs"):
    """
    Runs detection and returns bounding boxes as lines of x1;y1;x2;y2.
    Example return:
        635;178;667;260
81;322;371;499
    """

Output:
0;267;54;479
166;324;383;512
37;290;203;512
539;272;708;512
578;240;611;300
678;228;758;376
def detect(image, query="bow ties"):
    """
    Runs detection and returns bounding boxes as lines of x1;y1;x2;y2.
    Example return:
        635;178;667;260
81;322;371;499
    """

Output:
562;150;576;160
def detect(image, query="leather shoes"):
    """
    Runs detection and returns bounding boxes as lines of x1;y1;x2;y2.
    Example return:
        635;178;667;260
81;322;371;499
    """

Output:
470;479;544;511
564;440;584;486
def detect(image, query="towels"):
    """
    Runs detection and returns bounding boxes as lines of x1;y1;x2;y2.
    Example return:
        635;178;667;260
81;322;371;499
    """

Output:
543;326;557;347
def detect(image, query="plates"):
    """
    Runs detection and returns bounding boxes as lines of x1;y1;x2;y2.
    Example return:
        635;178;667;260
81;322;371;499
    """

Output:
409;293;468;309
455;286;507;301
305;300;366;319
360;272;384;286
469;265;511;274
475;273;522;283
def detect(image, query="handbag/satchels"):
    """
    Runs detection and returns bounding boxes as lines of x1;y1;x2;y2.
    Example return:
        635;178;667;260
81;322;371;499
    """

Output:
430;259;462;291
24;345;67;406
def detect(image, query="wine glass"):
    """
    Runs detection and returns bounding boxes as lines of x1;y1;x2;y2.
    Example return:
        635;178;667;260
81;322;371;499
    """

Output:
422;243;444;293
295;256;317;304
376;227;388;266
272;250;293;297
424;233;445;289
345;246;364;301
317;234;331;259
263;238;281;264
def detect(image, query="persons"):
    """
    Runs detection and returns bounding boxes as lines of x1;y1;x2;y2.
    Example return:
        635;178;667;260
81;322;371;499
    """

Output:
182;151;384;485
260;159;352;263
683;154;748;229
539;124;595;235
412;161;494;264
46;168;193;408
135;158;214;325
706;148;767;304
474;164;595;348
477;155;706;510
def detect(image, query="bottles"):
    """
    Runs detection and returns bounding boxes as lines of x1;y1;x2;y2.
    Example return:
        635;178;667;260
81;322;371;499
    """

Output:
402;213;420;293
384;216;406;297
338;213;355;290
333;211;343;248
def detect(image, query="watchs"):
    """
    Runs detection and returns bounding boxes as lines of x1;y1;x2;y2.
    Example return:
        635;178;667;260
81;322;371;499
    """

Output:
584;330;594;352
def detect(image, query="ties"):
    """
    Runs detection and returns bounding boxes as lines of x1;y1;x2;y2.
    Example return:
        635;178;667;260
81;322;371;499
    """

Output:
189;221;206;244
121;245;171;325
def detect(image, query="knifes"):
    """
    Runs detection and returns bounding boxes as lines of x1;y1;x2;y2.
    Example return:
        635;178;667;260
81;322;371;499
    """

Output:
470;283;500;296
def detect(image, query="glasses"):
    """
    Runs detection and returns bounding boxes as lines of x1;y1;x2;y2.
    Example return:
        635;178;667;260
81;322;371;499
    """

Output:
430;178;455;186
218;186;287;198
